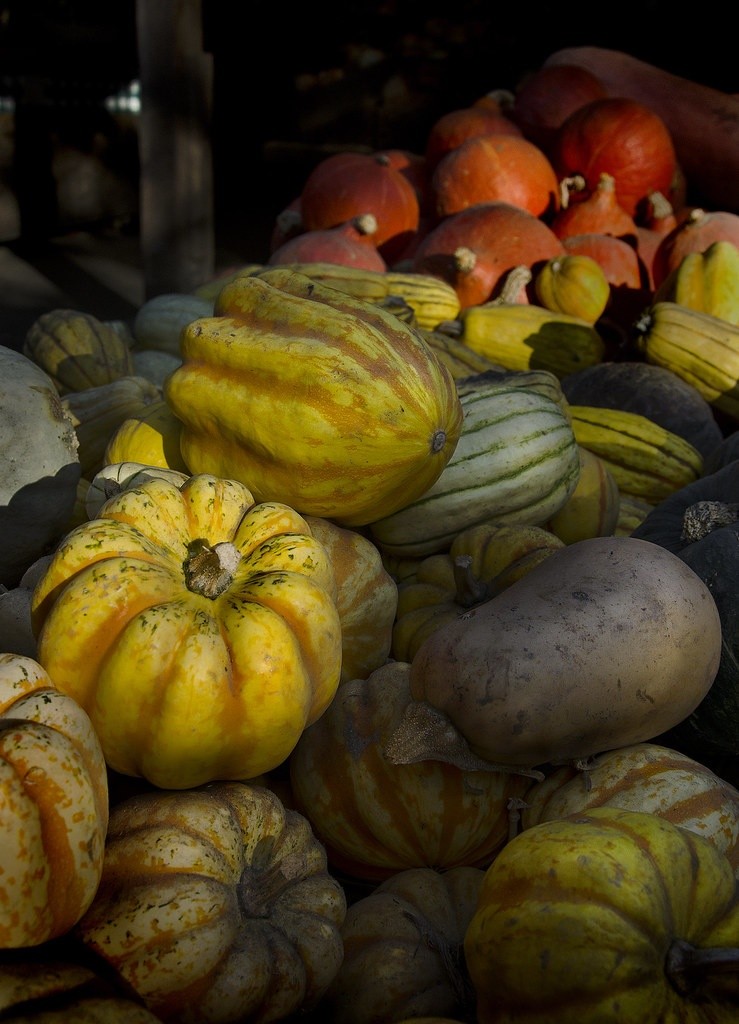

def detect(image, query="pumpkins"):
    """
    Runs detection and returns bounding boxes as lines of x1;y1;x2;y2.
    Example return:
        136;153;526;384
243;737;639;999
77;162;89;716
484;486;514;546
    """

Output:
0;47;739;1024
